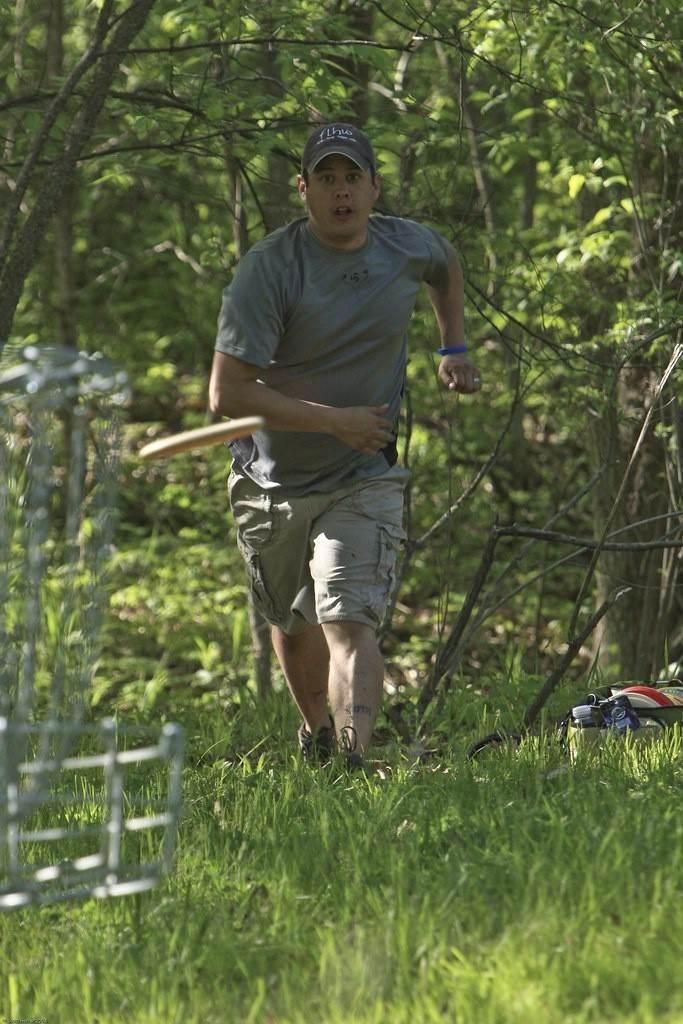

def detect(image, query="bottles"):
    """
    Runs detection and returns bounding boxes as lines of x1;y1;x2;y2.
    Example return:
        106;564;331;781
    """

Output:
568;705;600;776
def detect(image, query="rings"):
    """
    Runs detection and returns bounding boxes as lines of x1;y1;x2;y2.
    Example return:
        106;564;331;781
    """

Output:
474;378;479;382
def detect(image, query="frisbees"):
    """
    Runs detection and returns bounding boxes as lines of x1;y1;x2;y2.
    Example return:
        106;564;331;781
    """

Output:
137;411;265;463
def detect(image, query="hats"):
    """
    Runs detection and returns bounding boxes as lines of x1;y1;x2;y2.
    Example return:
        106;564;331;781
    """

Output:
299;123;376;175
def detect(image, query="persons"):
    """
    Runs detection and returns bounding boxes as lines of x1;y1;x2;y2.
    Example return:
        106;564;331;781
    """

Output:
208;122;484;780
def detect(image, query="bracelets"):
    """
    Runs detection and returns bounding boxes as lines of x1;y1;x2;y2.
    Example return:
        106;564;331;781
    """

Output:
438;345;467;354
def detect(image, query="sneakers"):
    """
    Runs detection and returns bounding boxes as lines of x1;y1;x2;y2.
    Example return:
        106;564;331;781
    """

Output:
334;726;369;781
298;712;338;782
